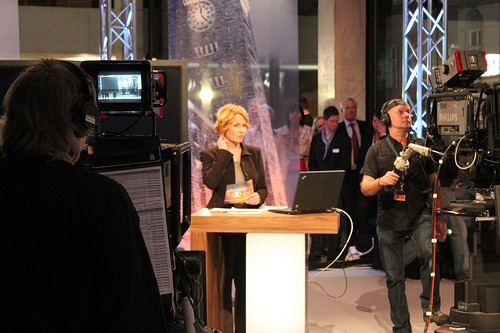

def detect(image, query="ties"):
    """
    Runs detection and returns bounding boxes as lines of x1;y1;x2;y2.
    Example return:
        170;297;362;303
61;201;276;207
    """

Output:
348;122;360;165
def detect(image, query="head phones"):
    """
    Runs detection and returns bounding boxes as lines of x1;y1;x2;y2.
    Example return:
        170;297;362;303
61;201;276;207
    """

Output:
60;60;98;137
381;100;414;126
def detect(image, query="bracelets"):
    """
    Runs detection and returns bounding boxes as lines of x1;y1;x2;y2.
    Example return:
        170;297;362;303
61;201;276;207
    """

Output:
378;178;383;188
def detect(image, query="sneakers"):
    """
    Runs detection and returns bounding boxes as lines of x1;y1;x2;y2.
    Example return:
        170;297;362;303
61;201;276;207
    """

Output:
422;309;450;326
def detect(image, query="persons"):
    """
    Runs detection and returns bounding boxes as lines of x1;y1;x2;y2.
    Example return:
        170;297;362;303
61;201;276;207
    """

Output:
360;99;449;333
439;152;478;309
244;98;390;262
199;103;268;333
0;60;169;333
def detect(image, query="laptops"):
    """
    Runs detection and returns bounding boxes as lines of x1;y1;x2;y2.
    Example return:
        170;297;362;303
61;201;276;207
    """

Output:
268;170;346;215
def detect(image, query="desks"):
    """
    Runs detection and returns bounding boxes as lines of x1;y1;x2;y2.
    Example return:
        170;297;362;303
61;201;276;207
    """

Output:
190;208;340;333
